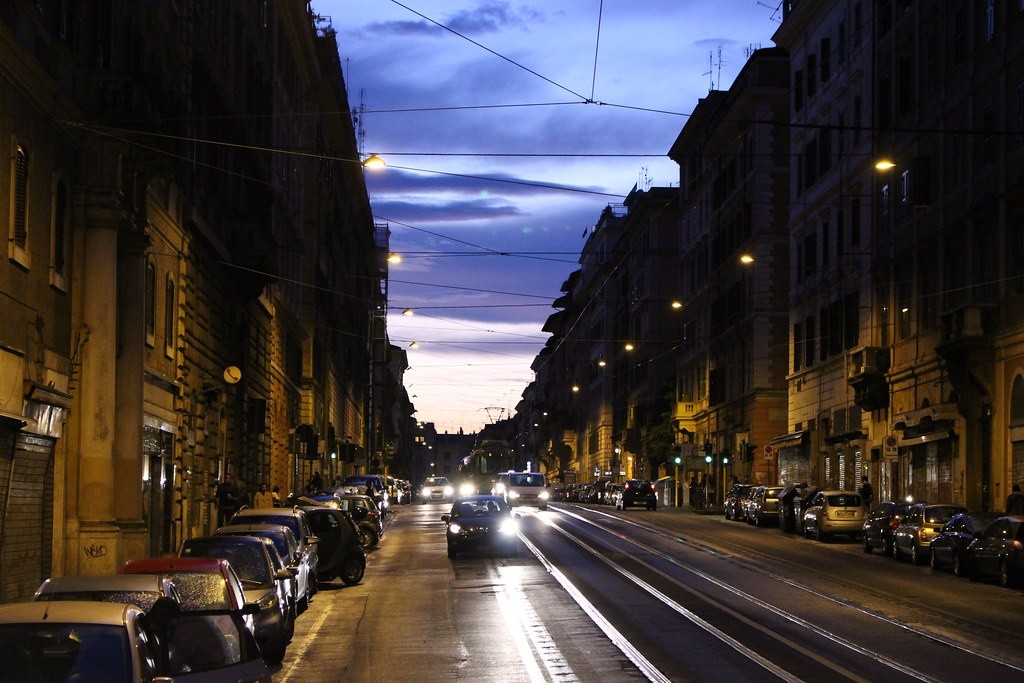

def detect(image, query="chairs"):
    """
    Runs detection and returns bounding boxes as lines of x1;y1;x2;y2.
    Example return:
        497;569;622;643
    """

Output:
68;634;129;683
0;639;43;683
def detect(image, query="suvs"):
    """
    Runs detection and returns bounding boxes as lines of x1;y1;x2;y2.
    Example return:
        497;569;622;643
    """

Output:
740;485;801;527
723;482;756;521
890;503;969;566
490;471;509;496
418;476;455;505
501;471;551;511
798;489;868;542
614;479;657;512
861;501;924;556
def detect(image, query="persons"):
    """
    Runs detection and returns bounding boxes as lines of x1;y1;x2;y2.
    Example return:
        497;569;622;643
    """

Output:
334;475;344;488
214;473;242;527
1006;484;1024;516
254;483;274;508
148;598;234;670
312;471;323;491
858;477;873;518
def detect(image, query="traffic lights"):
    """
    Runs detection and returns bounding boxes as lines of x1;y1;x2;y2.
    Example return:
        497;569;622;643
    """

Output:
704;442;713;464
721;451;730;465
673;446;682;465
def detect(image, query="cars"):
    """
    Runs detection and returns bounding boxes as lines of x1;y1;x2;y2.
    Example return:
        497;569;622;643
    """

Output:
0;601;276;683
440;494;522;559
964;513;1024;592
174;534;295;668
211;472;416;613
928;512;1009;578
116;555;257;646
28;572;182;614
547;479;623;506
